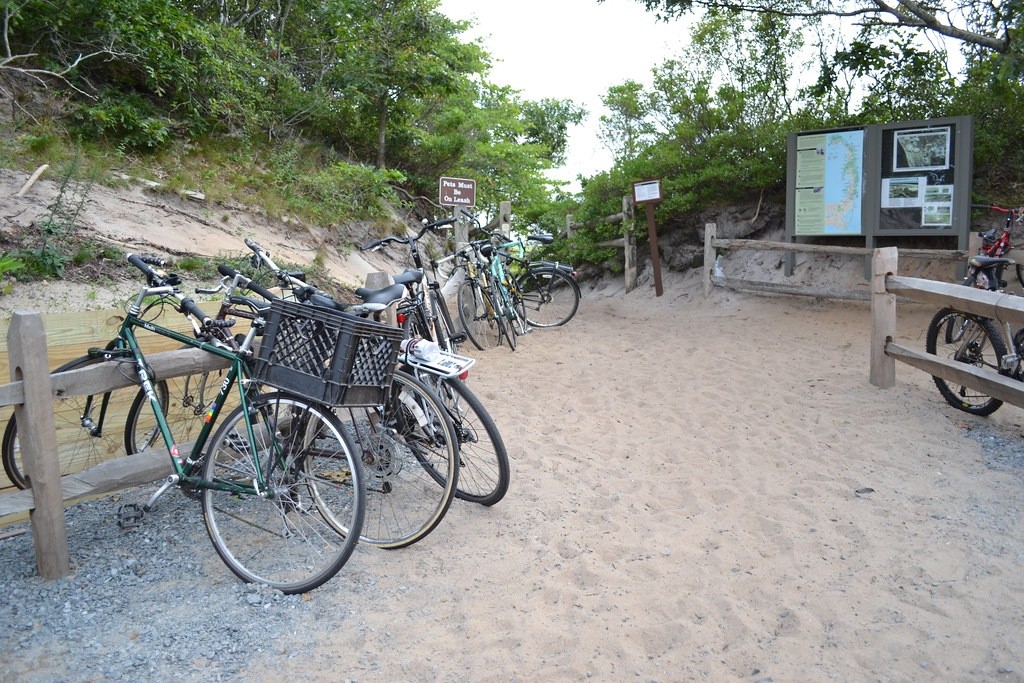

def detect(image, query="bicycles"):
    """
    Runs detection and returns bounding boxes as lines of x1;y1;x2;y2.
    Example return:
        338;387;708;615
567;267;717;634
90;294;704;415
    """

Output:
946;205;1024;345
243;237;510;506
480;238;523;351
3;263;456;550
430;237;500;350
1;252;365;592
359;216;465;368
461;210;582;328
925;257;1024;415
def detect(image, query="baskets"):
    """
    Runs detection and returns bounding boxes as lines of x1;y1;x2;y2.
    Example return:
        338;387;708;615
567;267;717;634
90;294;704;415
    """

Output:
252;297;404;407
